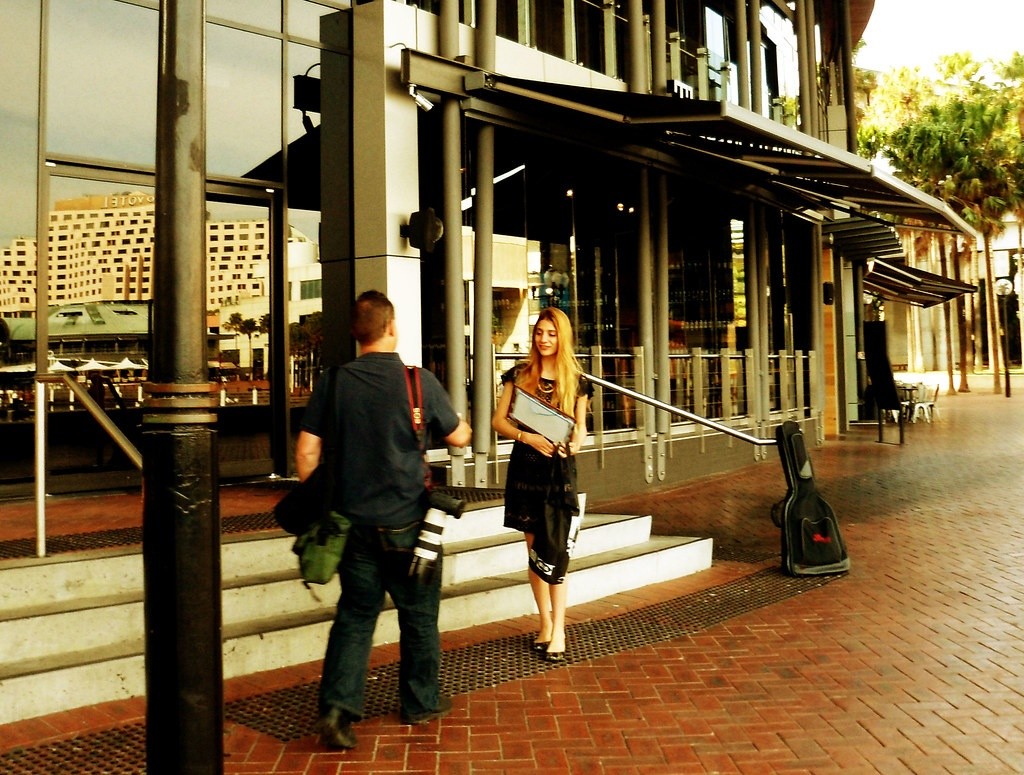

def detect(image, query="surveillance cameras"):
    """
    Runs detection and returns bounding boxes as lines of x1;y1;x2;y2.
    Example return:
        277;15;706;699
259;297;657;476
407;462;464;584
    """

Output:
414;94;434;112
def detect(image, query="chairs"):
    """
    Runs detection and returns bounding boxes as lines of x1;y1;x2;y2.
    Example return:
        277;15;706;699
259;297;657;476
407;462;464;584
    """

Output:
886;379;942;424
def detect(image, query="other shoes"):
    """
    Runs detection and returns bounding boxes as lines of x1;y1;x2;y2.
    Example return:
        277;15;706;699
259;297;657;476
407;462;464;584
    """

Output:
533;639;551;651
400;696;452;722
544;650;565;661
315;705;357;748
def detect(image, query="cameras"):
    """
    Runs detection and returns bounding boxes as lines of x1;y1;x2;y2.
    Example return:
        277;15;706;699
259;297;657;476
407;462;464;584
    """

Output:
406;491;466;592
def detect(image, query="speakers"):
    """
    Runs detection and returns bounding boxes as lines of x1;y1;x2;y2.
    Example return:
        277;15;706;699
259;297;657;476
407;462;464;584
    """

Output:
823;281;833;306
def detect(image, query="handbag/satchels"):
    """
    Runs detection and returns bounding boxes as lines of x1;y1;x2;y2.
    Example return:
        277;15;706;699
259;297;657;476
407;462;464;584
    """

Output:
293;512;350;585
529;443;579;584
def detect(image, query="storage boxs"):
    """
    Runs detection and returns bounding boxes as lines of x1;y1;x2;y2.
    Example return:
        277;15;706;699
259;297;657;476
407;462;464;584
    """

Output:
770;420;851;579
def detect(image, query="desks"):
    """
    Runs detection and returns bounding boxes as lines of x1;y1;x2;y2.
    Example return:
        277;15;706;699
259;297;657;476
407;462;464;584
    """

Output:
898;388;922;422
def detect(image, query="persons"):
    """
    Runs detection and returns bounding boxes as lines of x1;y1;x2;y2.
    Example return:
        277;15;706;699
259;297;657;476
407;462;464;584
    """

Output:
488;305;604;660
293;288;477;750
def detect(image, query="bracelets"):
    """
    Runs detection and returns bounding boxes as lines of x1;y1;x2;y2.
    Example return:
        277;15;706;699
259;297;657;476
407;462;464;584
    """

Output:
516;432;522;440
